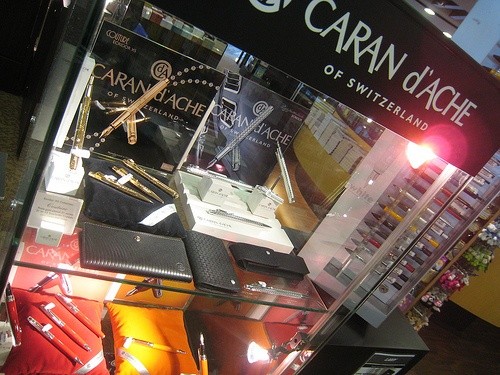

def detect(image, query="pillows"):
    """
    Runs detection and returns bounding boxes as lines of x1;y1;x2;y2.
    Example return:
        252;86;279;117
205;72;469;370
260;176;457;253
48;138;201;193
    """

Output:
6;286;110;375
82;158;187;239
200;313;278;375
105;301;198;375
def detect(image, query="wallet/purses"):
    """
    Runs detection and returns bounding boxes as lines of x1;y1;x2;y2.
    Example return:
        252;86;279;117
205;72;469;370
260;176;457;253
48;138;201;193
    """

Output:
183;229;241;296
80;221;191;282
229;243;310;276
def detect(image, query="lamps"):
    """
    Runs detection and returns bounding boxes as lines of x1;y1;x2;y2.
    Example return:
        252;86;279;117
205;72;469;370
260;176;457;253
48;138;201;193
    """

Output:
247;340;294;363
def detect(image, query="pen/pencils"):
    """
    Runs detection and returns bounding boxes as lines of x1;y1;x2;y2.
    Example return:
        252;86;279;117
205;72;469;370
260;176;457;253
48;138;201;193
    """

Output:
39;303;93;353
87;170;154;203
55;290;106;340
122;158;179;199
62;273;71;293
274;140;296;204
211;209;273;228
198;330;208;375
124;276;156;298
6;282;22;345
70;71;95;169
124;335;187;355
28;271;59;293
243;284;311;300
204;105;275;170
111;165;165;204
154;279;162;297
98;77;170;139
27;316;86;366
343;150;499;290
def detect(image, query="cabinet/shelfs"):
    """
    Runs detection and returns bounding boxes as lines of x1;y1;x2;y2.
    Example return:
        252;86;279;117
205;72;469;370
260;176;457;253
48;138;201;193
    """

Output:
297;129;499;329
140;6;228;69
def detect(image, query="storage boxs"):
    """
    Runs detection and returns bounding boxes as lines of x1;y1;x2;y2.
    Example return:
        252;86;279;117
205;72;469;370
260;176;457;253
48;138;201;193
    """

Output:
45;150;86;197
